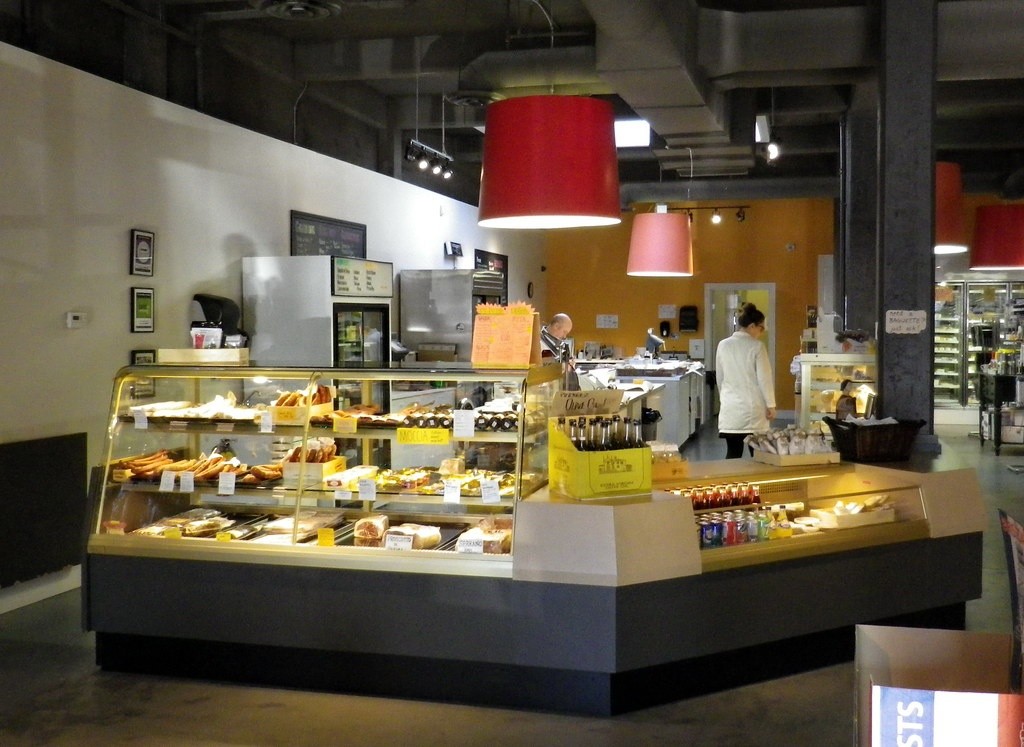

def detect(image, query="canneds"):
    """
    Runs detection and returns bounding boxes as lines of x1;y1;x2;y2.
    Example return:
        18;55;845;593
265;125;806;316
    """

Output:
694;510;748;549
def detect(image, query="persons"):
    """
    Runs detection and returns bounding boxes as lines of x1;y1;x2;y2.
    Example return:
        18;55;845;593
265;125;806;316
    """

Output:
716;302;775;459
539;313;575;370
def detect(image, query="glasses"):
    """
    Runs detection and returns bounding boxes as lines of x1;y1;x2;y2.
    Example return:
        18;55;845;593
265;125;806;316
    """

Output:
755;324;765;332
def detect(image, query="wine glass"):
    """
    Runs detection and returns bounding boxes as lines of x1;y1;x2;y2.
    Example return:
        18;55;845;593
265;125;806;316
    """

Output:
998;354;1022;375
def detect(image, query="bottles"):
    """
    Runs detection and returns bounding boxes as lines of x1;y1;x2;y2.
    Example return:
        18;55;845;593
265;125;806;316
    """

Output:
694;505;792;550
665;481;762;518
558;415;645;451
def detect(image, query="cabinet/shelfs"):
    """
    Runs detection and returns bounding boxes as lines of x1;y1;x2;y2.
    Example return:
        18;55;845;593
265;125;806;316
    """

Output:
615;366;705;453
978;373;1024;455
801;353;877;440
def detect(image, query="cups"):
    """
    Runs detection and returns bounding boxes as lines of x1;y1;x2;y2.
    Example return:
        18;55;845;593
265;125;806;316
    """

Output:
980;364;989;373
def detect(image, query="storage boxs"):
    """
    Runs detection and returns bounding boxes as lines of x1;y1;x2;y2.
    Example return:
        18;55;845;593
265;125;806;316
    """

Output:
158;348;249;367
854;625;1024;746
267;402;333;424
652;457;689;483
810;507;894;529
823;416;927;462
282;456;347;483
754;450;840;467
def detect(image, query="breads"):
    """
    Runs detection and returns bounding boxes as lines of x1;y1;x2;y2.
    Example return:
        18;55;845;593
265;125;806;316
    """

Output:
311;403;428;424
811;365;877;412
117;449;282;483
128;391;267;423
274;385;331;406
289;444;337;463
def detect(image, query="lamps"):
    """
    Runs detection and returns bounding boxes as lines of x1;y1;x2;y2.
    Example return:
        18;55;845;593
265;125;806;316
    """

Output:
626;202;694;277
406;74;456;180
667;206;751;224
